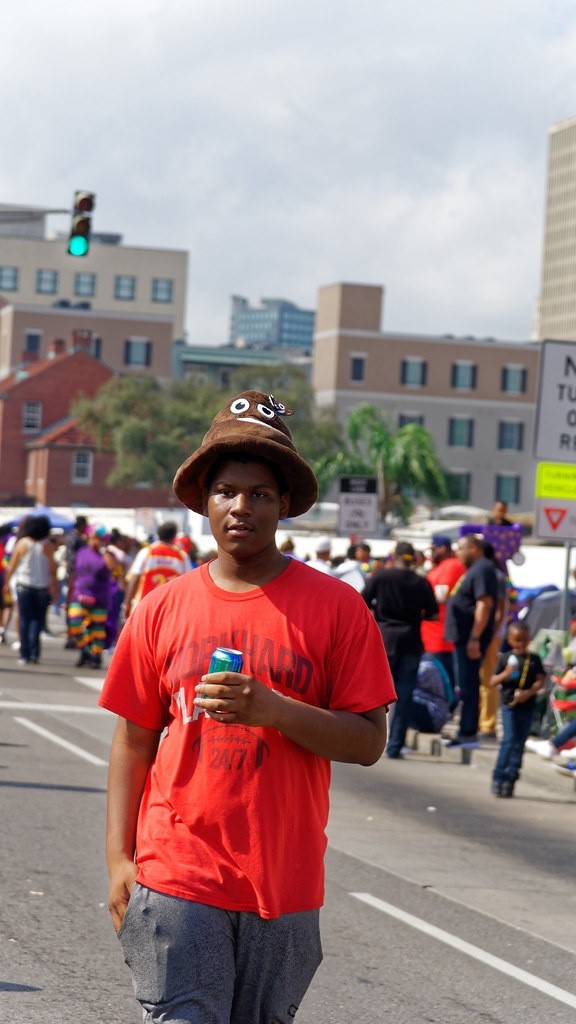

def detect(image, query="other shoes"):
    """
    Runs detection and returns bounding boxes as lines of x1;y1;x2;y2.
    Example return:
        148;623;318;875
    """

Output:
446;736;479;748
491;781;513;799
560;747;576;759
524;739;557;760
553;762;576;778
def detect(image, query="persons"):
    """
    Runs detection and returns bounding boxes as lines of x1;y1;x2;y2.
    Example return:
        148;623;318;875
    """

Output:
123;522;192;615
6;516;54;664
276;533;507;595
99;391;399;1024
421;535;499;749
361;541;438;758
57;511;132;669
491;622;576;797
476;498;527;578
408;643;455;733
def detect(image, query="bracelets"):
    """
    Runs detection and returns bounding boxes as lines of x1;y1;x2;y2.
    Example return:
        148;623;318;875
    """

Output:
471;637;480;643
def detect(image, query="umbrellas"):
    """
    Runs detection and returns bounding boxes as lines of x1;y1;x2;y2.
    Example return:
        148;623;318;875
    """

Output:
0;505;76;531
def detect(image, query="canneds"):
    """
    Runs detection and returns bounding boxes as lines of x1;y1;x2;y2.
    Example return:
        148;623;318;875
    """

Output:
201;646;243;700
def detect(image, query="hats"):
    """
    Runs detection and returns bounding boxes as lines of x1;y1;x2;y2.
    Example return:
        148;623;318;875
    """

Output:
397;542;414;556
173;390;319;518
425;537;450;550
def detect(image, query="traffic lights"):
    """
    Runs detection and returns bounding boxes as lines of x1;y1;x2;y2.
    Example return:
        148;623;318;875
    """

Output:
67;191;92;258
